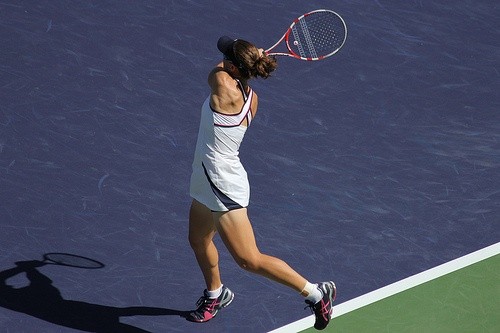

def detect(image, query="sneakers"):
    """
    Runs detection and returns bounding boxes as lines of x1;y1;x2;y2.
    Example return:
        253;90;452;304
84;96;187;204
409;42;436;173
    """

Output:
186;284;234;323
304;280;336;330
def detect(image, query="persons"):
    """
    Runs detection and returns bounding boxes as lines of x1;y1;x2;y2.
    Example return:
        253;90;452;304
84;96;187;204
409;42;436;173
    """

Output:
186;36;335;330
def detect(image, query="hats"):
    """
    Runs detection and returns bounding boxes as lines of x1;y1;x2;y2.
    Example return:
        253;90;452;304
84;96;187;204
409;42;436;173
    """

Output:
217;34;250;78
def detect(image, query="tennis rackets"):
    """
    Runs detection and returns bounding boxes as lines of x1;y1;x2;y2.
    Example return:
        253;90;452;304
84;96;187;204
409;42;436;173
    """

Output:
265;9;348;61
